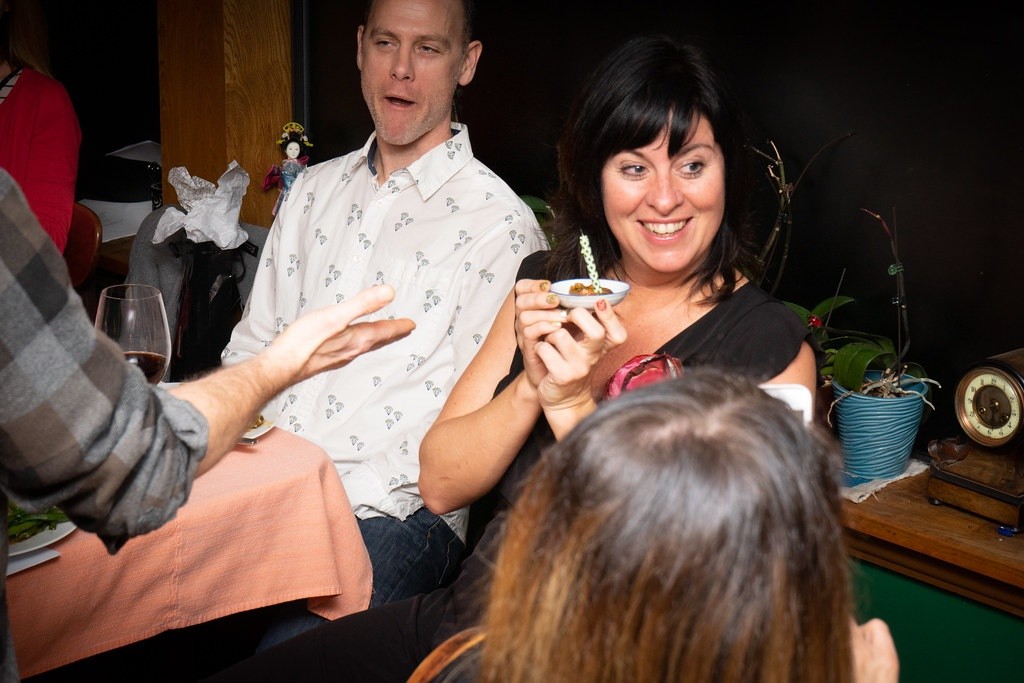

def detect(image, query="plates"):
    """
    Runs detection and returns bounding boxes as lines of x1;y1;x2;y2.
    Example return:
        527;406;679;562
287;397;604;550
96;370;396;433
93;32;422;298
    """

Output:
7;501;76;557
243;408;279;439
550;278;629;310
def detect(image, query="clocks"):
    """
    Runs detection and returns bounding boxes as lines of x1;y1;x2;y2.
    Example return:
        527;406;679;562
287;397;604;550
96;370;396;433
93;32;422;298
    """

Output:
922;347;1024;534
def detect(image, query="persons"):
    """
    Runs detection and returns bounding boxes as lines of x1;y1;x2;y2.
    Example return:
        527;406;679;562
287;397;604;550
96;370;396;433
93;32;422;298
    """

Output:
216;49;822;681
0;174;416;683
409;362;904;681
0;40;79;263
210;2;553;670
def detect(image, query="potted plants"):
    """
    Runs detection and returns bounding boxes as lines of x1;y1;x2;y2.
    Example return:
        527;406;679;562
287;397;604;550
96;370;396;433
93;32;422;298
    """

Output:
826;206;942;481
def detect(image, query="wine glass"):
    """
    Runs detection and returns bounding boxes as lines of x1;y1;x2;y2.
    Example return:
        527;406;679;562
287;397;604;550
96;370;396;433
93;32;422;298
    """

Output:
96;285;171;385
